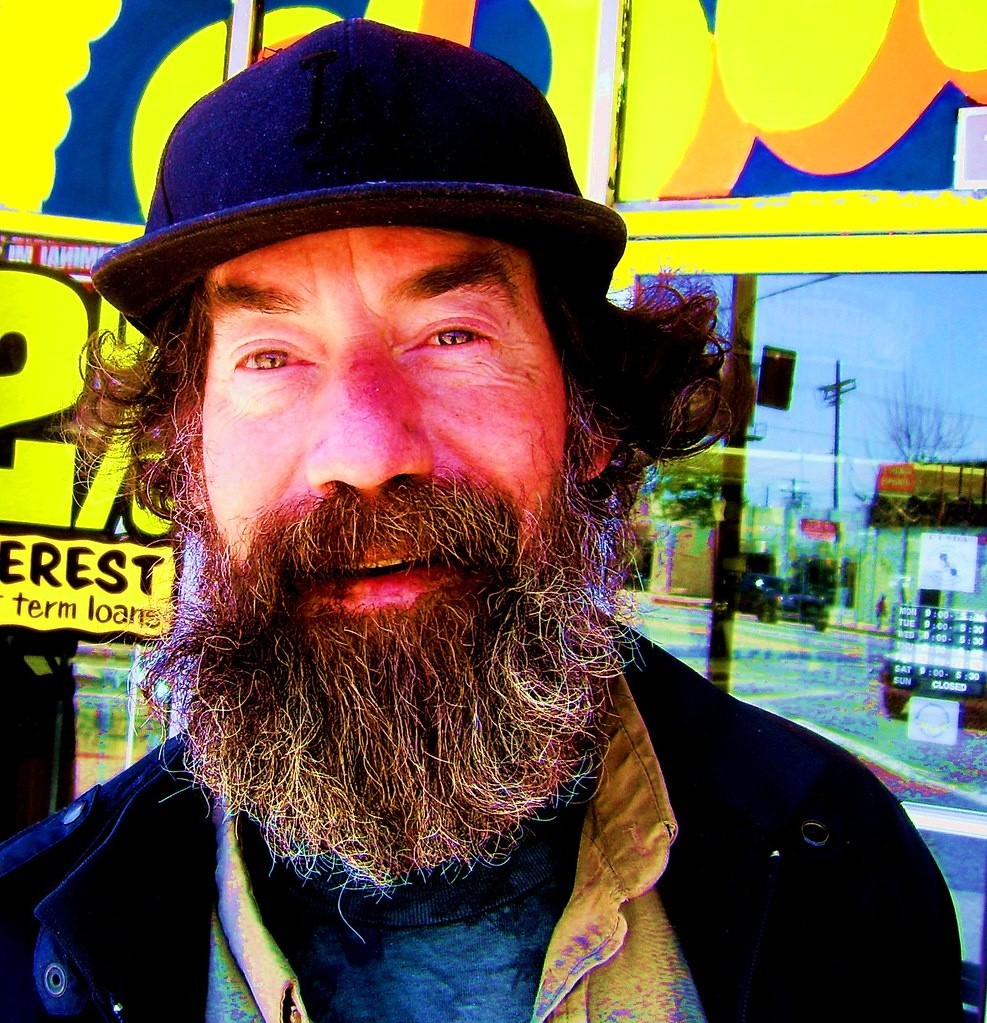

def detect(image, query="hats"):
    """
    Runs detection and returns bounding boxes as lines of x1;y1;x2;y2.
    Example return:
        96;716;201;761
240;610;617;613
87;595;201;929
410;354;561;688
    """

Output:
87;16;627;349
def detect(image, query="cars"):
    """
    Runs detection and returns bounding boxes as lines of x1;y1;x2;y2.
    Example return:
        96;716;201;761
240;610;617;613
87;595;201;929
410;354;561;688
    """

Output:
734;572;830;632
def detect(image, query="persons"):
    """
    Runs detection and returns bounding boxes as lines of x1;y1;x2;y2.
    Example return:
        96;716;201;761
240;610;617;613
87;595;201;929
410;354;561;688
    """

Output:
0;18;965;1022
